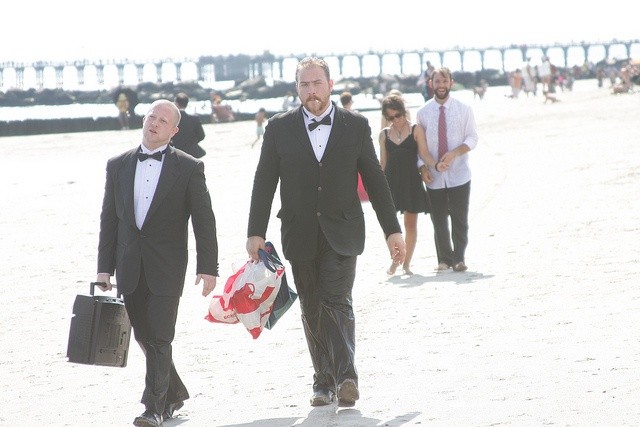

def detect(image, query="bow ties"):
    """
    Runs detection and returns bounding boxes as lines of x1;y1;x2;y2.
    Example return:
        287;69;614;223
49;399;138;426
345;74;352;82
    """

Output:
302;107;333;132
137;146;167;161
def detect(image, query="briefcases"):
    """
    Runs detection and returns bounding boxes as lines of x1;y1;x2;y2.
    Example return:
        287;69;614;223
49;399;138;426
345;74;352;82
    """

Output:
65;280;132;367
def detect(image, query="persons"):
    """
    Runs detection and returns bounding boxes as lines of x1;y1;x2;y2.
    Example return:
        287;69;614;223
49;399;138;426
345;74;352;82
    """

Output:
95;99;219;427
340;91;360;115
117;93;130;129
417;61;433;103
379;95;447;275
425;66;436;98
473;56;640;103
251;107;266;148
169;92;206;158
417;68;478;270
245;57;406;406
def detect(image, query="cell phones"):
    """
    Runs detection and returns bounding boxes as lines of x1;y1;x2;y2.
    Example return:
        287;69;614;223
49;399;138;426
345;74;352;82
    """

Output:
387;251;400;274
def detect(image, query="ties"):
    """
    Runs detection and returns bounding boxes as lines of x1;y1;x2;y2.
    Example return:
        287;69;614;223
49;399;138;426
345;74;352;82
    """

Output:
438;106;448;158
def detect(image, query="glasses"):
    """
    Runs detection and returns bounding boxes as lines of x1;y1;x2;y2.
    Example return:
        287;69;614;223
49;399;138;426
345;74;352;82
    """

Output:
388;112;403;120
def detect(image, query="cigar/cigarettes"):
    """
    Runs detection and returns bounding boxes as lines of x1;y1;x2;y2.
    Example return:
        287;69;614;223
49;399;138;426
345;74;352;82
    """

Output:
315;99;319;103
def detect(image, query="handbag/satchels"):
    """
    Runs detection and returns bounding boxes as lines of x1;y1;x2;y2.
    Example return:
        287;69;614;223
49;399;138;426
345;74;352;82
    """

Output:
204;258;285;339
258;241;298;330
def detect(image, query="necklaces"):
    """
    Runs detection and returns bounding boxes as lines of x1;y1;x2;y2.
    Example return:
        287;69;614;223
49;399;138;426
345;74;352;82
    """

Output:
393;123;405;142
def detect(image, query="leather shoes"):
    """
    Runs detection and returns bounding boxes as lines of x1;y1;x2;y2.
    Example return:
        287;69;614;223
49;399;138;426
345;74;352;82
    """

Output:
337;379;359;407
133;409;164;426
453;261;467;270
162;401;184;422
434;264;449;271
311;389;336;405
